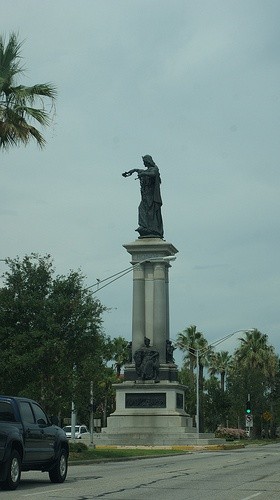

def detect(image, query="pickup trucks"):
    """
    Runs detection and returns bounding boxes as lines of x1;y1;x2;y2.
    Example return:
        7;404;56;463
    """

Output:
0;396;70;489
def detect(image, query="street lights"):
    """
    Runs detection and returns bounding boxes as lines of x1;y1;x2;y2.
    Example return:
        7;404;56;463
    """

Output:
69;255;175;445
170;329;253;429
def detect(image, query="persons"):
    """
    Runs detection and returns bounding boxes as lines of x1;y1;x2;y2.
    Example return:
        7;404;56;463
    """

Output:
126;341;132;363
166;340;176;364
122;154;166;238
132;336;160;383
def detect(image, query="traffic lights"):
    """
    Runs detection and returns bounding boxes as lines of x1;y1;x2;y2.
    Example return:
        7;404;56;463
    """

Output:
246;401;252;415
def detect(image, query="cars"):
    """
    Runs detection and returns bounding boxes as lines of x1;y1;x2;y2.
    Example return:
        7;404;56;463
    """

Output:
61;425;90;439
